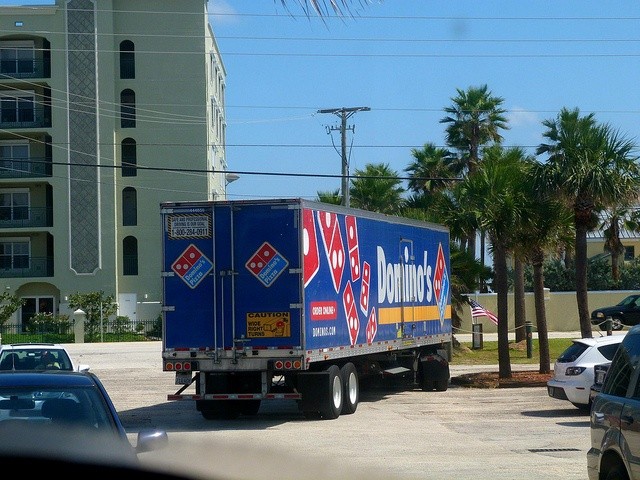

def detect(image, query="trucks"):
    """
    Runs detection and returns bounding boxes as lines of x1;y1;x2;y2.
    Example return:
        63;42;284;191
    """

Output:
159;197;453;421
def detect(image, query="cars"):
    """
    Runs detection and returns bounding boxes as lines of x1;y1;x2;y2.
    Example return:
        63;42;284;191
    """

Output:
547;334;624;411
0;343;90;373
587;324;640;480
590;295;639;330
1;369;168;466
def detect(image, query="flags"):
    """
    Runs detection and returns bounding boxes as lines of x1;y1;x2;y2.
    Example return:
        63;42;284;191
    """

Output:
468;297;498;326
